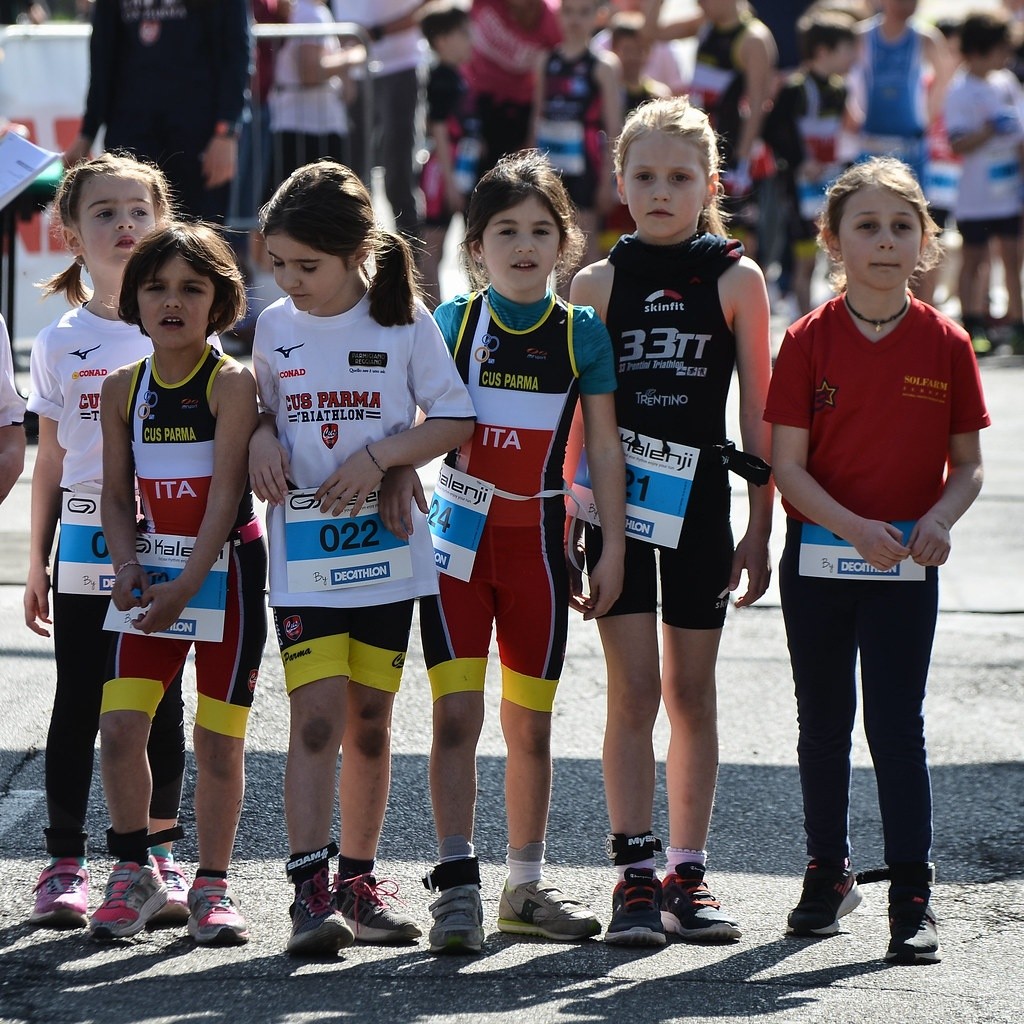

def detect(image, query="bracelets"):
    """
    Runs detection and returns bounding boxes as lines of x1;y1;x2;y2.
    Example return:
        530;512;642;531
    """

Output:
116;560;140;576
366;445;386;473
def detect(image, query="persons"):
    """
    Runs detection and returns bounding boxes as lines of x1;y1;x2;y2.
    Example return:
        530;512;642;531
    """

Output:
22;151;223;926
570;95;776;947
89;222;268;943
0;311;27;508
63;0;1024;357
762;158;992;962
248;161;477;953
382;149;627;951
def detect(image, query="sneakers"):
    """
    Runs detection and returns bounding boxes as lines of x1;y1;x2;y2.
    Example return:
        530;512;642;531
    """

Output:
149;847;191;920
886;884;943;963
496;878;602;939
659;861;743;939
428;889;484;954
31;857;88;923
330;872;424;941
286;868;355;954
90;854;167;938
786;859;864;936
186;877;249;944
606;867;666;946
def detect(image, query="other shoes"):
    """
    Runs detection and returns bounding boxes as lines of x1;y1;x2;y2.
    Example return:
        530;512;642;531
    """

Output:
959;311;1024;360
